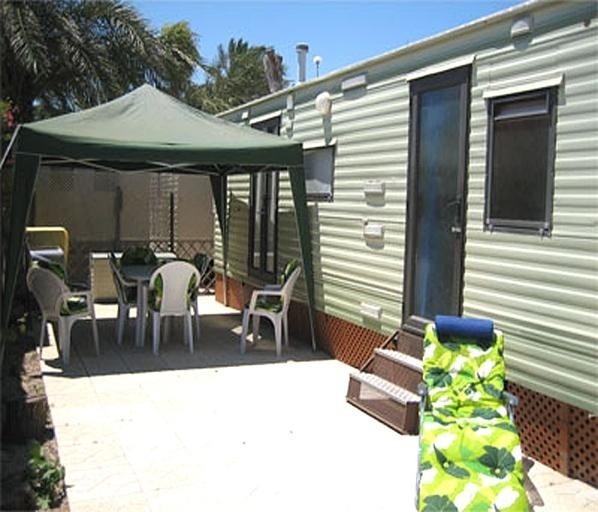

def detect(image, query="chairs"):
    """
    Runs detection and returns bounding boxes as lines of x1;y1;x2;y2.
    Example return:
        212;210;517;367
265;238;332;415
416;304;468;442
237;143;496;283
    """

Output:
108;242;215;355
417;315;531;511
240;259;299;357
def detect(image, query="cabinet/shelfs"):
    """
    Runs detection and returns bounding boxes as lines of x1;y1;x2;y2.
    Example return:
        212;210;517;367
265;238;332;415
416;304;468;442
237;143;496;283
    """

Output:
90;251;177;302
25;255;102;363
